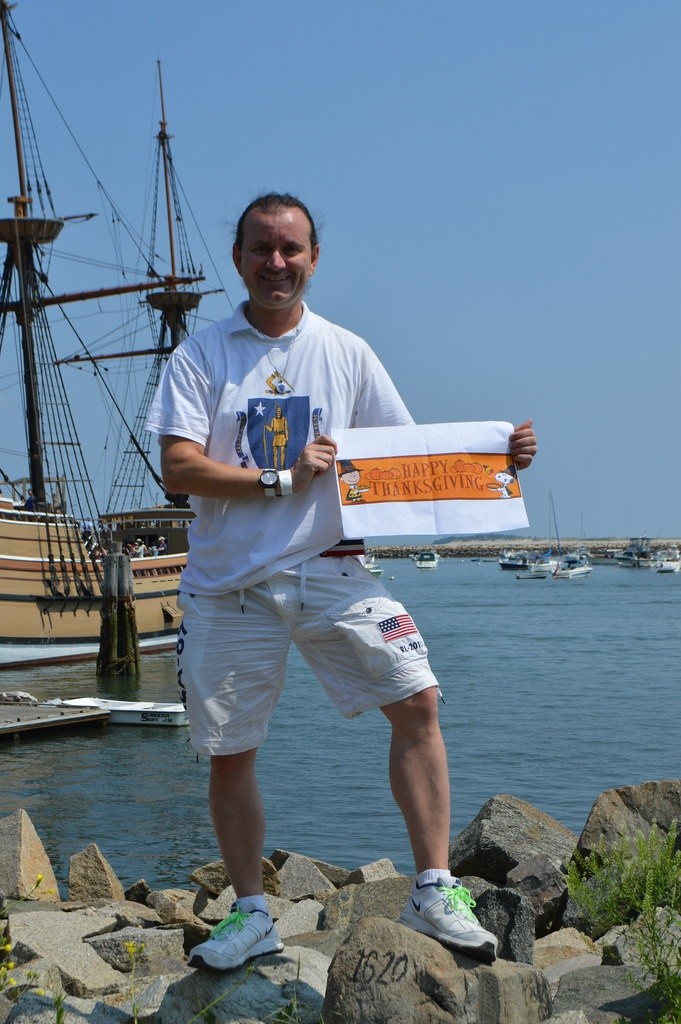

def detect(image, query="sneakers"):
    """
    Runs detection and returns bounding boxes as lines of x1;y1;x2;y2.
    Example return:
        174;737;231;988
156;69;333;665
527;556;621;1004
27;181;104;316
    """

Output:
187;901;284;971
399;876;498;963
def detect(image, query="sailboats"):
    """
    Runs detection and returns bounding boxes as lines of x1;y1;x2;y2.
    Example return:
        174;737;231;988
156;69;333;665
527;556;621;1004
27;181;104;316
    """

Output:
530;489;563;575
89;58;191;556
0;0;189;674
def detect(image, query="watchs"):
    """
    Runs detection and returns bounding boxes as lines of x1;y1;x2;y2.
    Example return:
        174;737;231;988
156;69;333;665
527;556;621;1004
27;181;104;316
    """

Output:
260;468;279;497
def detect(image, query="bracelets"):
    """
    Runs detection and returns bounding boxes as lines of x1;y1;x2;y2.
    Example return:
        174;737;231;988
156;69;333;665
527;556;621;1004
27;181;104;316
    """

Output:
277;470;293;495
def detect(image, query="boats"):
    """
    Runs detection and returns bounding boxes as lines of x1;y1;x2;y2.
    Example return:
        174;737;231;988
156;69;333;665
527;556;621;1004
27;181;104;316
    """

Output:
550;566;594;580
416;551;437;569
352;554;384;578
561;545;591;571
365;563;379;570
516;573;547;579
588;548;624;565
409;554;441;561
499;546;531;569
655;543;681;574
614;535;660;567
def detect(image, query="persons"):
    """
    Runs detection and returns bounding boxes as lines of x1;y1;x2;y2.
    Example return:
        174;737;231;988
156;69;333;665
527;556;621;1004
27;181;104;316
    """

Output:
142;192;538;971
25;489;70;524
80;517;167;558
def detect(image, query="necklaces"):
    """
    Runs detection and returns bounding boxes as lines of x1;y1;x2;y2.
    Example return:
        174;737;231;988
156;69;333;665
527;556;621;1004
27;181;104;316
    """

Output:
248;315;297;394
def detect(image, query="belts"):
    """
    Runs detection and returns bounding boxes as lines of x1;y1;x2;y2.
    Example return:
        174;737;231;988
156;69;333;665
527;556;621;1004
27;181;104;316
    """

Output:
319;539;366;556
